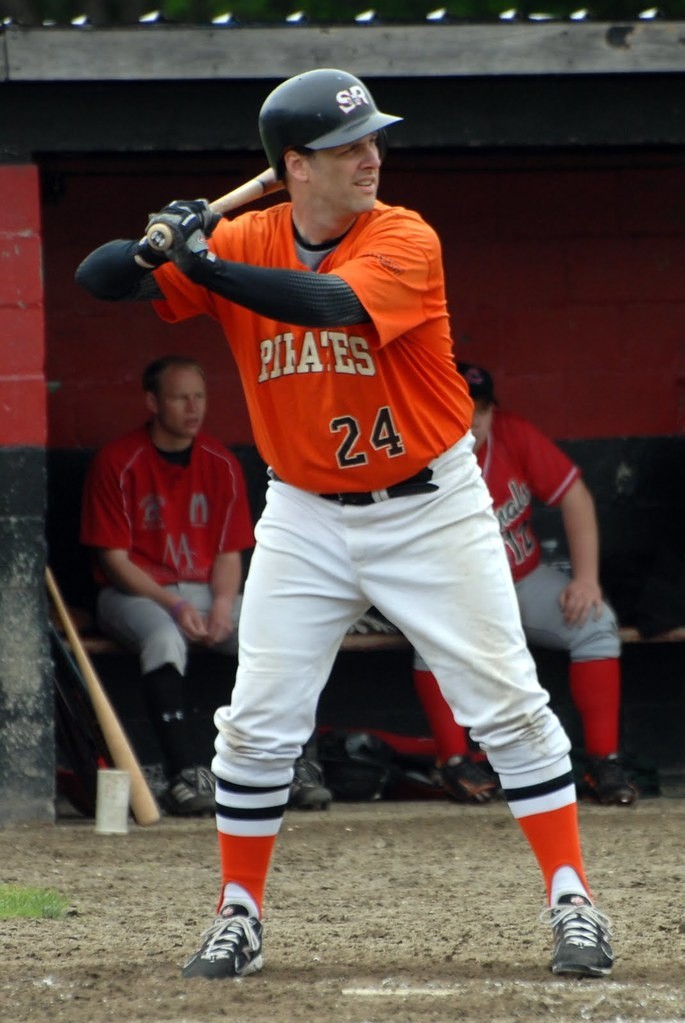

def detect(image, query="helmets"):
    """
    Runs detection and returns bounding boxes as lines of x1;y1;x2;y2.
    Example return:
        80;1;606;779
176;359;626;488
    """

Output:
258;68;405;181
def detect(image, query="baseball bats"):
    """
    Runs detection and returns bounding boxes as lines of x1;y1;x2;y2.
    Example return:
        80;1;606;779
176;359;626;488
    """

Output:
44;565;161;827
146;166;286;252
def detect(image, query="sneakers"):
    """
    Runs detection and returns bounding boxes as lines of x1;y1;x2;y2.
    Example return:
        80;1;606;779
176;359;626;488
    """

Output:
538;894;615;976
166;764;215;813
182;905;265;980
582;753;638;804
290;758;331;811
431;755;496;802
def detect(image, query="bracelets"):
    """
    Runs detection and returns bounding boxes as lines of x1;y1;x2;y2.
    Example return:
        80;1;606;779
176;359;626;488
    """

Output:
170;598;188;619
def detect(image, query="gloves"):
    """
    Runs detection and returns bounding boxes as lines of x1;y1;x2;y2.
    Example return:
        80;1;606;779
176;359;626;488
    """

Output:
137;198;223;267
144;199;216;285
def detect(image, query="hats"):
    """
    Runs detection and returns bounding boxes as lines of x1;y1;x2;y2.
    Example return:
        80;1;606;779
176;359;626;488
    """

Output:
457;363;500;406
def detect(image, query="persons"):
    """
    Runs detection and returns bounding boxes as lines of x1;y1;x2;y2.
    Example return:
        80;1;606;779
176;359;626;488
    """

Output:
411;365;636;809
76;69;614;980
80;354;332;821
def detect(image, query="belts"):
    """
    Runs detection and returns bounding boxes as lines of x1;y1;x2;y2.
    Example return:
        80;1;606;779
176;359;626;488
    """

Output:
273;466;440;504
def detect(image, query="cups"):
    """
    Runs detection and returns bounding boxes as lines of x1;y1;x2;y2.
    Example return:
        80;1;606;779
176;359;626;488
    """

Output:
95;770;131;832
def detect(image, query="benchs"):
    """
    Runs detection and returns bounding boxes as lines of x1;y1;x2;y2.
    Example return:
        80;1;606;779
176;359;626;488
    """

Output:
56;619;684;820
